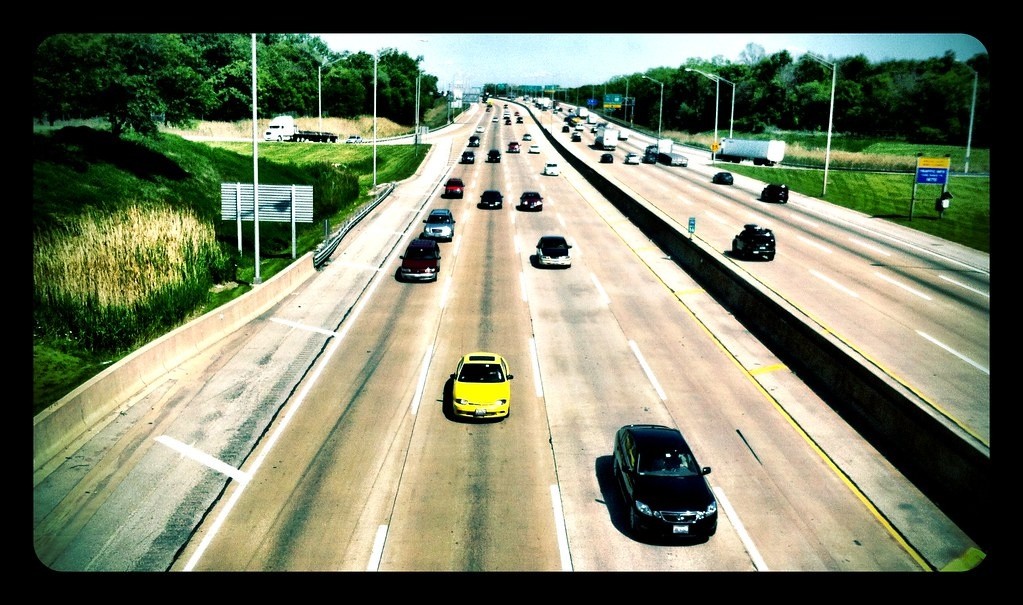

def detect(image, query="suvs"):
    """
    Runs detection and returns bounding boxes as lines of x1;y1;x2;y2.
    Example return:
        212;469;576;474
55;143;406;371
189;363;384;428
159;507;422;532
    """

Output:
732;224;777;261
762;184;790;204
346;135;363;144
422;209;455;241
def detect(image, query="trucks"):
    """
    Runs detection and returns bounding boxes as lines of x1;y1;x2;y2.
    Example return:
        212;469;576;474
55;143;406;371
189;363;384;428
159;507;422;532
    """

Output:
657;138;688;167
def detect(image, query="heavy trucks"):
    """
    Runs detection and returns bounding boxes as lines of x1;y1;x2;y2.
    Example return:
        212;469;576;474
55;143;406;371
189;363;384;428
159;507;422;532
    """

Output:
263;116;338;143
714;138;786;166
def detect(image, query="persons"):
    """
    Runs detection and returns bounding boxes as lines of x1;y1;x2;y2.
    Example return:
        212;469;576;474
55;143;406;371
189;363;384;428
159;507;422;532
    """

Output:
558;244;564;248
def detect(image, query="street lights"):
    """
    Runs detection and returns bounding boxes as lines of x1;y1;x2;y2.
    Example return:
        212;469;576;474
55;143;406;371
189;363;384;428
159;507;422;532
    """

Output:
621;75;629;124
796;49;837;196
374;37;428;188
642;75;663;138
319;56;348;131
686;68;720;164
708;74;735;139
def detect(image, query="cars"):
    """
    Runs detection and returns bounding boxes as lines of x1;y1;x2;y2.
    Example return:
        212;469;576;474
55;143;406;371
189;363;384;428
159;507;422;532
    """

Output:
544;162;559;176
536;236;573;267
712;172;733;184
451;353;514;422
481;191;504;210
444;178;465;198
614;425;716;544
534;97;658;165
461;94;541;163
520;191;544;212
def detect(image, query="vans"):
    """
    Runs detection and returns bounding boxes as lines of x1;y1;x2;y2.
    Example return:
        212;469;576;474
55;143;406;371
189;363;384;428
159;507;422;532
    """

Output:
400;239;442;282
